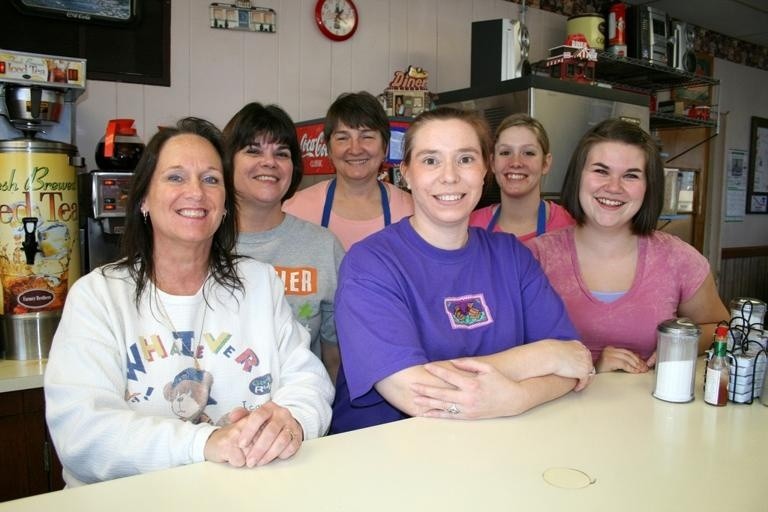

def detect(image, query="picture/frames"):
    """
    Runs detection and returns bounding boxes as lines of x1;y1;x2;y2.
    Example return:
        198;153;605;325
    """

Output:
669;54;715;122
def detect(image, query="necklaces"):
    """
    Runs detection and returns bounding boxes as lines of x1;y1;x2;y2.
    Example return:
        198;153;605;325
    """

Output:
151;274;211;372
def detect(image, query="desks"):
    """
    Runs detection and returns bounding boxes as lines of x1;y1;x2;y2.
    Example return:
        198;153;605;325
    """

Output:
0;348;768;512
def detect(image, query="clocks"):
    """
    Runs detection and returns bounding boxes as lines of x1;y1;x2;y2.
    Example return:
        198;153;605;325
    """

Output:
314;0;359;42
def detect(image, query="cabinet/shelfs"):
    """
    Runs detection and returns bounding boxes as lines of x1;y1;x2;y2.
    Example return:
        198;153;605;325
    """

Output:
0;358;65;502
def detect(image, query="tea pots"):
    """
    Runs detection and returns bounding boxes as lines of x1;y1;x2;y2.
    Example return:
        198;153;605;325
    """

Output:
95;118;144;171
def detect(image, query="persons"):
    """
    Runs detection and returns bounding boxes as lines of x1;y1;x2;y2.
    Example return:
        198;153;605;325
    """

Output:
217;102;350;383
523;116;733;374
466;113;581;245
280;90;415;255
327;106;597;444
42;116;336;491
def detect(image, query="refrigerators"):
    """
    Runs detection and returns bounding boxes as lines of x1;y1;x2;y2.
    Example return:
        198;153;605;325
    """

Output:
294;116;335;190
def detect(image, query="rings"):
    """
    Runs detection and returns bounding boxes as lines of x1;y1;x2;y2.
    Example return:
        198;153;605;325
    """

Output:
587;365;598;380
445;401;460;416
282;426;295;440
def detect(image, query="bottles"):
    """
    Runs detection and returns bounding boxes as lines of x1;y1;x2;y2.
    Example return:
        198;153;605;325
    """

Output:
650;317;702;404
726;297;766;330
705;326;729;407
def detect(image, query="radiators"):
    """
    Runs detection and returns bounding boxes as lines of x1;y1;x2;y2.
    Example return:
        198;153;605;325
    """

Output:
718;244;768;307
